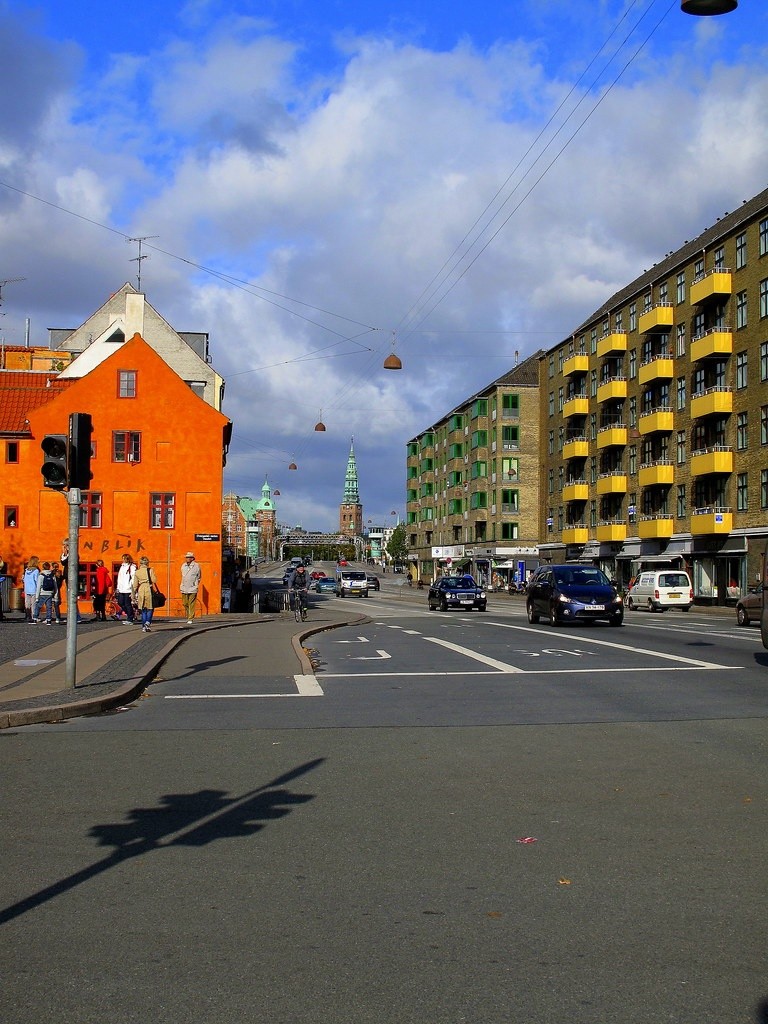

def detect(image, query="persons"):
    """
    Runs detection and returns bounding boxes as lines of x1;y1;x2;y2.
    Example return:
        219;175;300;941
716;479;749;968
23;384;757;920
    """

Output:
407;571;413;584
180;552;201;624
89;560;109;621
131;556;157;633
115;554;136;626
229;571;253;610
287;563;311;618
0;538;82;626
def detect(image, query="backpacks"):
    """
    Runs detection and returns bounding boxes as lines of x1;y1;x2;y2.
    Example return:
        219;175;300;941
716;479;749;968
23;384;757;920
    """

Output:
41;572;54;591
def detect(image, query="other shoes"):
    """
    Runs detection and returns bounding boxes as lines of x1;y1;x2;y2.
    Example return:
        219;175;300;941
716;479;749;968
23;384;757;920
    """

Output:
63;619;67;622
100;615;106;621
123;621;133;626
90;616;101;622
187;621;192;624
111;615;118;620
55;620;59;623
142;628;146;633
46;621;51;626
144;623;151;632
42;620;47;624
303;612;307;619
28;620;37;625
76;619;81;623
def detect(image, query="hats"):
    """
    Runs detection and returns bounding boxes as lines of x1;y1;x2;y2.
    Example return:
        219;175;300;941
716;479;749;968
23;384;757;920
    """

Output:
184;552;195;558
297;563;304;568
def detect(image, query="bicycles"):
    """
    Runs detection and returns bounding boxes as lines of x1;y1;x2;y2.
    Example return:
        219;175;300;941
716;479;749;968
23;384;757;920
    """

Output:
289;589;308;623
408;578;412;587
104;591;143;623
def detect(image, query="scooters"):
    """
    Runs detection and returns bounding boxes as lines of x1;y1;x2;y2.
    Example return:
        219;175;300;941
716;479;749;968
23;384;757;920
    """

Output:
508;580;527;596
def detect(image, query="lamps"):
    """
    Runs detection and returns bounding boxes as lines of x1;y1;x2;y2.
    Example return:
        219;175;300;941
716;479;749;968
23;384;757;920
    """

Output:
315;408;325;431
383;331;402;369
264;494;270;506
273;483;280;495
508;457;516;475
288;453;297;469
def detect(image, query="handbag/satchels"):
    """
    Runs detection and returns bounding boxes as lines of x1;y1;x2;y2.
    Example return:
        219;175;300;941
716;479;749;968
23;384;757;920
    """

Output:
105;573;112;587
152;592;166;607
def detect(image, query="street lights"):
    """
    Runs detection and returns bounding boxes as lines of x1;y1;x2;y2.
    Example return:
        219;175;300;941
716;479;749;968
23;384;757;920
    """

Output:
391;511;400;526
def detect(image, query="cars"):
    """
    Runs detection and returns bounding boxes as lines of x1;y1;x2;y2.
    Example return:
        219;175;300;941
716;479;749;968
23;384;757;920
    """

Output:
282;555;313;586
428;576;487;613
526;565;625;627
366;576;380;592
736;580;764;627
339;559;348;567
309;571;338;593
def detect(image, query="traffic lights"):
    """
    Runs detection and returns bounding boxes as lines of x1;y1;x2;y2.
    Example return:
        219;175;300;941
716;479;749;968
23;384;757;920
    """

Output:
67;412;94;490
41;434;66;487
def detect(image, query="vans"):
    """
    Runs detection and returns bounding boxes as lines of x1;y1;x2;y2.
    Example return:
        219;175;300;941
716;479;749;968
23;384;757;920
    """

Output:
627;570;694;614
335;569;368;599
